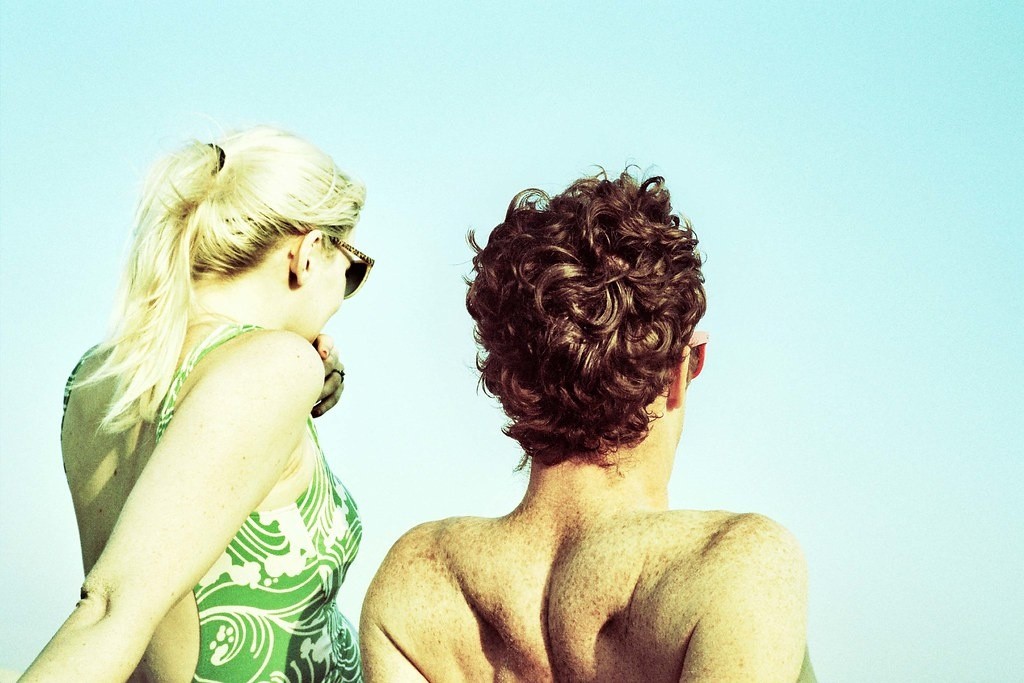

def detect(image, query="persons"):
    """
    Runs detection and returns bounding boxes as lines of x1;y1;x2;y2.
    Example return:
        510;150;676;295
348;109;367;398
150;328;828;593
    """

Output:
12;126;365;682
359;172;822;683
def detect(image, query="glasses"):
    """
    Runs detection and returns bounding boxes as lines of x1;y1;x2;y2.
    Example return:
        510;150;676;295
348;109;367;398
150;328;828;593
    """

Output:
329;237;375;299
688;331;707;379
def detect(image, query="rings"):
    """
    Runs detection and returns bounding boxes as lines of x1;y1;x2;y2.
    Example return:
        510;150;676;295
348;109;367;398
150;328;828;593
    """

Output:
331;368;345;383
